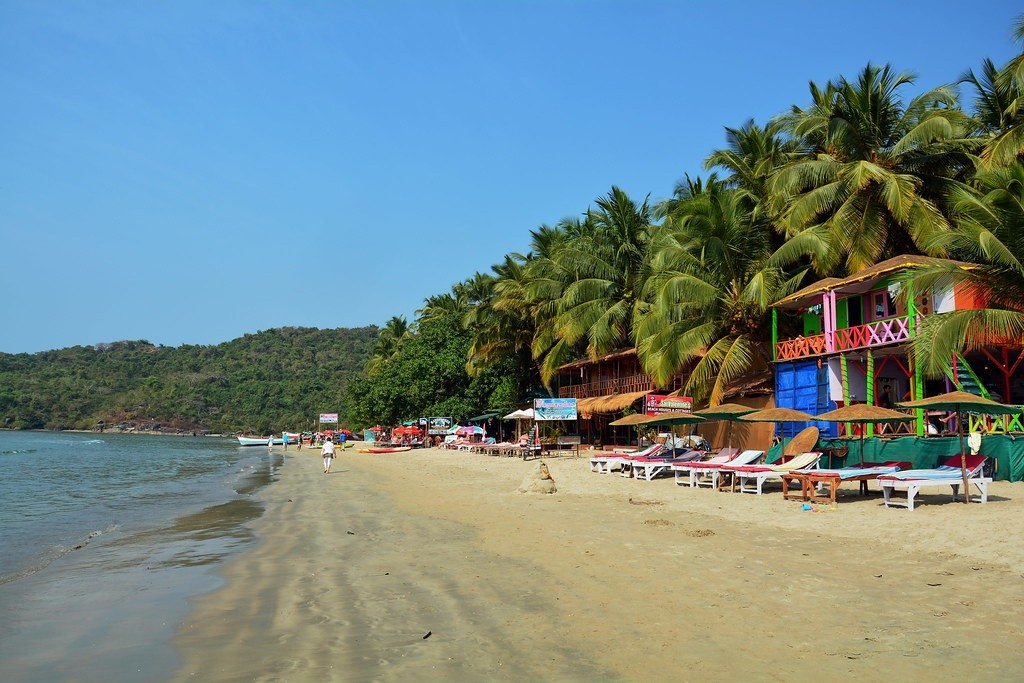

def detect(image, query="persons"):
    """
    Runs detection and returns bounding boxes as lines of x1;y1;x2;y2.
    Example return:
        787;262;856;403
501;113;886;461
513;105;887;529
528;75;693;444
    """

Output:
378;434;433;447
297;434;303;451
310;431;346;473
519;432;528;456
699;434;705;449
283;433;288;451
435;435;441;446
268;435;273;452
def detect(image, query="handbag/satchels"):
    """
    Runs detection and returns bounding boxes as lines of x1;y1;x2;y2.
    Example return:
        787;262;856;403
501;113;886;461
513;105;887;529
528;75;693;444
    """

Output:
310;439;313;442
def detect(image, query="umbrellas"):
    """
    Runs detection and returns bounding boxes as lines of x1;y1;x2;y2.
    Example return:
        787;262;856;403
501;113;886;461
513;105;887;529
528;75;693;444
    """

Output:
638;412;707;476
894;391;1022;503
503;407;535;438
368;426;382;432
692;404;760;462
322;430;335;435
339;430;352;435
393;424;425;439
738;408;822;464
810;404;918;494
609;414;652;450
450;423;488;443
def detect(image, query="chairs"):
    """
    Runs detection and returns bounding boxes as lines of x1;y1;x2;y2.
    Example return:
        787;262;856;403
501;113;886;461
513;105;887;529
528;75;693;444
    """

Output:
439;436;993;511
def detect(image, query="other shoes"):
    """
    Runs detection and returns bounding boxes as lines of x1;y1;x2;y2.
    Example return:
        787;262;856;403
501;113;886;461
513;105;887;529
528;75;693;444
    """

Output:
324;470;329;473
297;449;301;451
340;449;345;452
284;449;287;451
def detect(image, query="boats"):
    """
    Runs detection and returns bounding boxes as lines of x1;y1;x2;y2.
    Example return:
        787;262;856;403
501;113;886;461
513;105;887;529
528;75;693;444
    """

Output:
355;446;411;454
305;443;355;449
235;436;299;447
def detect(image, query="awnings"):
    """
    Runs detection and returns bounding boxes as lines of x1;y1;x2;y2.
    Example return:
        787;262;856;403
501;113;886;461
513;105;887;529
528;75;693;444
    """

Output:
575;390;653;413
470;414;500;421
